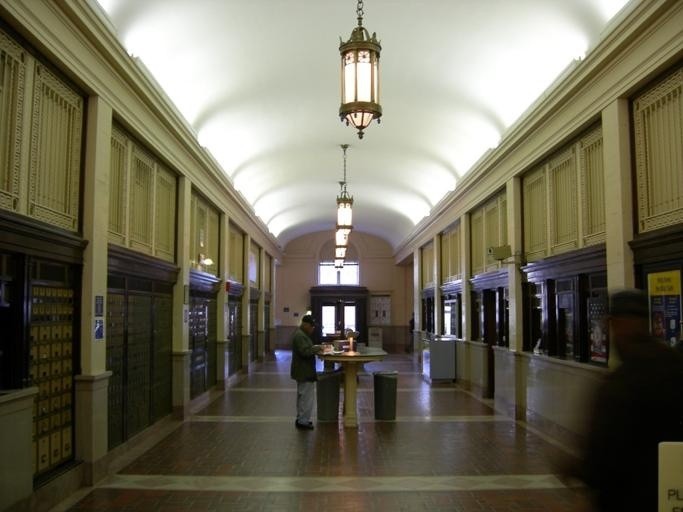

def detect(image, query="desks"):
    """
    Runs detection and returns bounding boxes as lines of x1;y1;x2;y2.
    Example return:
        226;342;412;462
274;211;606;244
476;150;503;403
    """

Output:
317;341;387;428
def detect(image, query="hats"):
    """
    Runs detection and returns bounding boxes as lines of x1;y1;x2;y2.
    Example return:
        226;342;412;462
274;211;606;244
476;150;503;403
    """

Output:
302;314;319;326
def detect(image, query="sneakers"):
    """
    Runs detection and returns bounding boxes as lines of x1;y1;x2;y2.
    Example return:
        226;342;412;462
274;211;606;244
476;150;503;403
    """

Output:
295;418;313;430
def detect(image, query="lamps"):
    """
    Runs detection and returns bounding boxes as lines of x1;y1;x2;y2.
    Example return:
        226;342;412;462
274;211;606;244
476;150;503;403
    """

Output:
337;0;381;139
334;144;353;229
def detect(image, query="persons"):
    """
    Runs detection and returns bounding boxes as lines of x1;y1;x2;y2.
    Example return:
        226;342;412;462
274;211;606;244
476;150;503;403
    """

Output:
556;288;683;512
290;315;321;429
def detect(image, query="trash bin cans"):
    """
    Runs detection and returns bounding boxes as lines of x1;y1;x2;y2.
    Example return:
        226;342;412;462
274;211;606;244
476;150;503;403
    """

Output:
368;327;382;350
372;372;398;420
317;373;341;421
